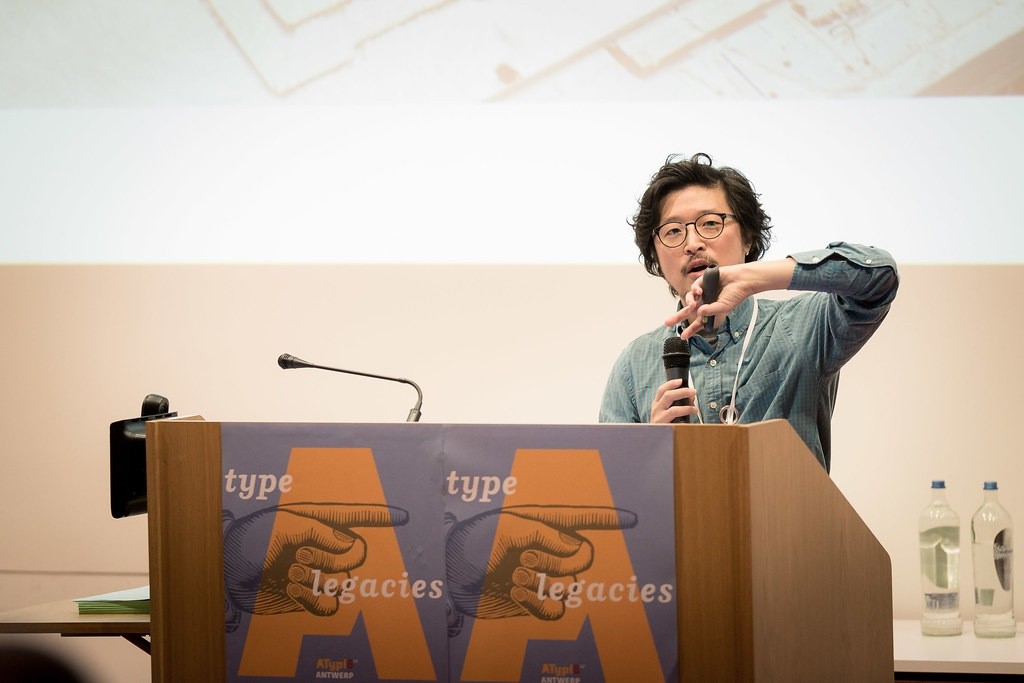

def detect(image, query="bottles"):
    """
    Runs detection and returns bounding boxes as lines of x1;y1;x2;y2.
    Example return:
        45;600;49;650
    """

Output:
919;480;963;635
971;481;1016;637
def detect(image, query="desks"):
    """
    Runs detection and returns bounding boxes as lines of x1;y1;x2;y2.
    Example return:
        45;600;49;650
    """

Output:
0;600;150;659
894;618;1024;683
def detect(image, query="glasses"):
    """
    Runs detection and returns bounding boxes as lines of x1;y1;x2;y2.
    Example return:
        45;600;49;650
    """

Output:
651;213;739;248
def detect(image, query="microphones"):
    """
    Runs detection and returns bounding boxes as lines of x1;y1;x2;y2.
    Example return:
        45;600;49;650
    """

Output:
278;353;423;423
661;337;691;423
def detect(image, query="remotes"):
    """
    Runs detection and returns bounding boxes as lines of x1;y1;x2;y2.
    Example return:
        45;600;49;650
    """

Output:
703;262;720;333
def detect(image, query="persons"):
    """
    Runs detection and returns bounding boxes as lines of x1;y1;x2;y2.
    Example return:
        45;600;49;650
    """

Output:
597;152;899;474
